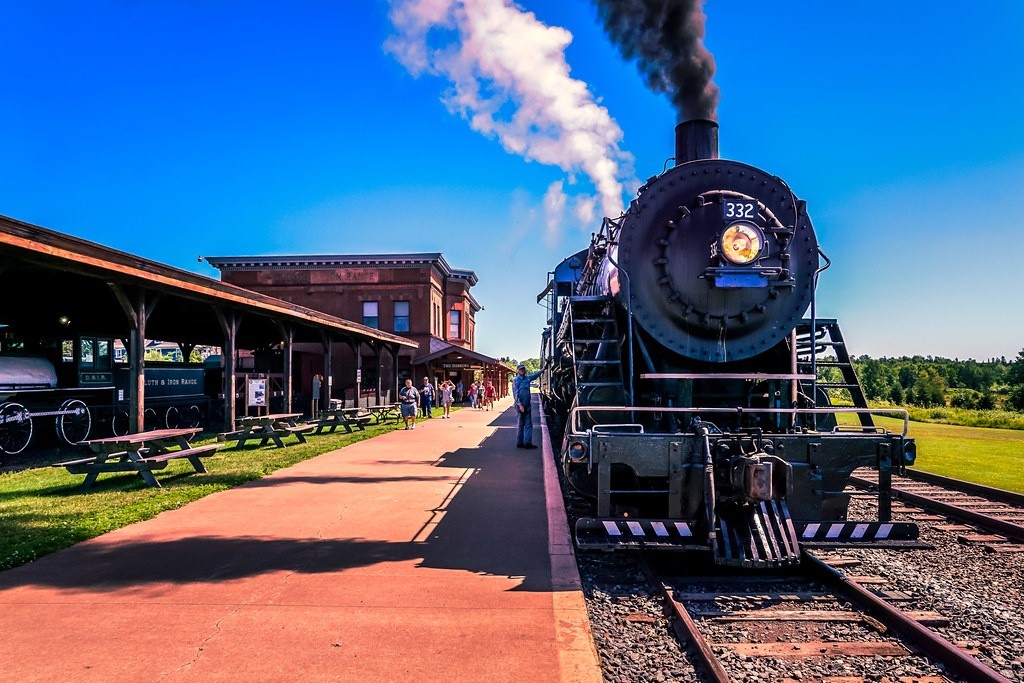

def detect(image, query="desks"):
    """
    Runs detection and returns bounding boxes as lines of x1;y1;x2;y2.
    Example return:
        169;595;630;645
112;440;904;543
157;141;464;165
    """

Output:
365;405;395;424
76;427;208;493
315;408;362;434
234;413;307;450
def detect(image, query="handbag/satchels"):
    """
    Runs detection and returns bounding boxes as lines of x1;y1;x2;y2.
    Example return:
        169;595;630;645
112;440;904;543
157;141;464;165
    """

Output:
450;397;454;402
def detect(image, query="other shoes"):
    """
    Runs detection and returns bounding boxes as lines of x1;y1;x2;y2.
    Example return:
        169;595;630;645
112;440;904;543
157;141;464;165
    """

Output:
486;408;488;411
447;416;449;418
442;416;446;419
428;414;432;418
405;427;409;430
411;425;415;429
525;443;537;449
516;442;525;448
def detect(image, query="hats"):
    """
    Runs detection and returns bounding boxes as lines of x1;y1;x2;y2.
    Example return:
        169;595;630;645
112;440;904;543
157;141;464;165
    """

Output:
517;365;525;370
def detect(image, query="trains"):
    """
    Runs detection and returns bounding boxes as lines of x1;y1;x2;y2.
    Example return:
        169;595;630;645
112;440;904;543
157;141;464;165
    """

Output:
0;294;296;465
536;119;935;566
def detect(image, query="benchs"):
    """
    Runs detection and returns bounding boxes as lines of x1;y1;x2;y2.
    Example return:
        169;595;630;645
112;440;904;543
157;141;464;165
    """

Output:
218;423;319;440
307;408;422;426
51;444;226;474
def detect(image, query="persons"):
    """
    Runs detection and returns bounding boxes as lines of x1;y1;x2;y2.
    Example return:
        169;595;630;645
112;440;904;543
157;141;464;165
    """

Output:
511;365;544;448
467;381;485;410
399;379;420;430
456;380;463;403
418;376;436;418
439;380;456;419
483;382;496;411
